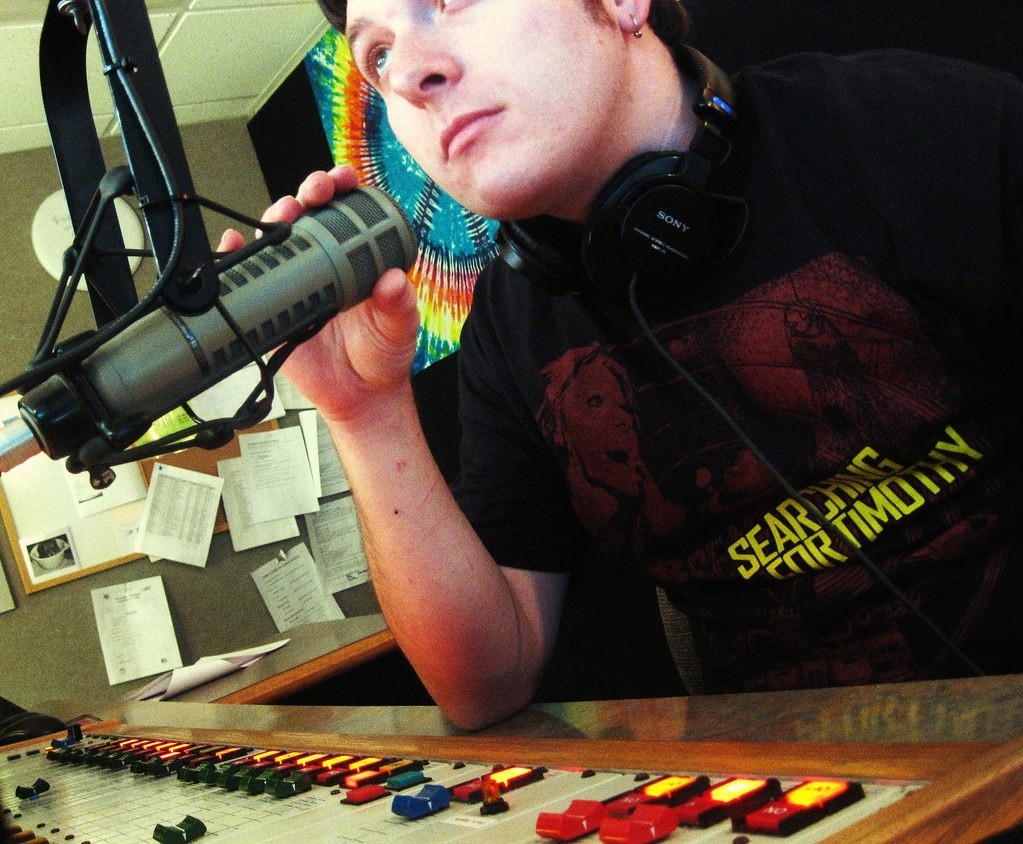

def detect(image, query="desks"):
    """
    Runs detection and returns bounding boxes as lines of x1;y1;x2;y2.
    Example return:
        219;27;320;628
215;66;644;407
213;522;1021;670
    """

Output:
165;616;398;705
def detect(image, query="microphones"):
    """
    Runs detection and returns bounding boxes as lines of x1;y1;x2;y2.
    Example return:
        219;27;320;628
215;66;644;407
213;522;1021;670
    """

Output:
17;186;419;461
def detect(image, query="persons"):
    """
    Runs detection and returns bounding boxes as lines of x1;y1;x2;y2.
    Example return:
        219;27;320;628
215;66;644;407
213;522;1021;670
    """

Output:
221;0;1023;733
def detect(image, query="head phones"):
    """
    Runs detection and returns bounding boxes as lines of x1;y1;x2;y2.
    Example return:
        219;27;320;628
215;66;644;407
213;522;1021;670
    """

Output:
491;42;740;303
